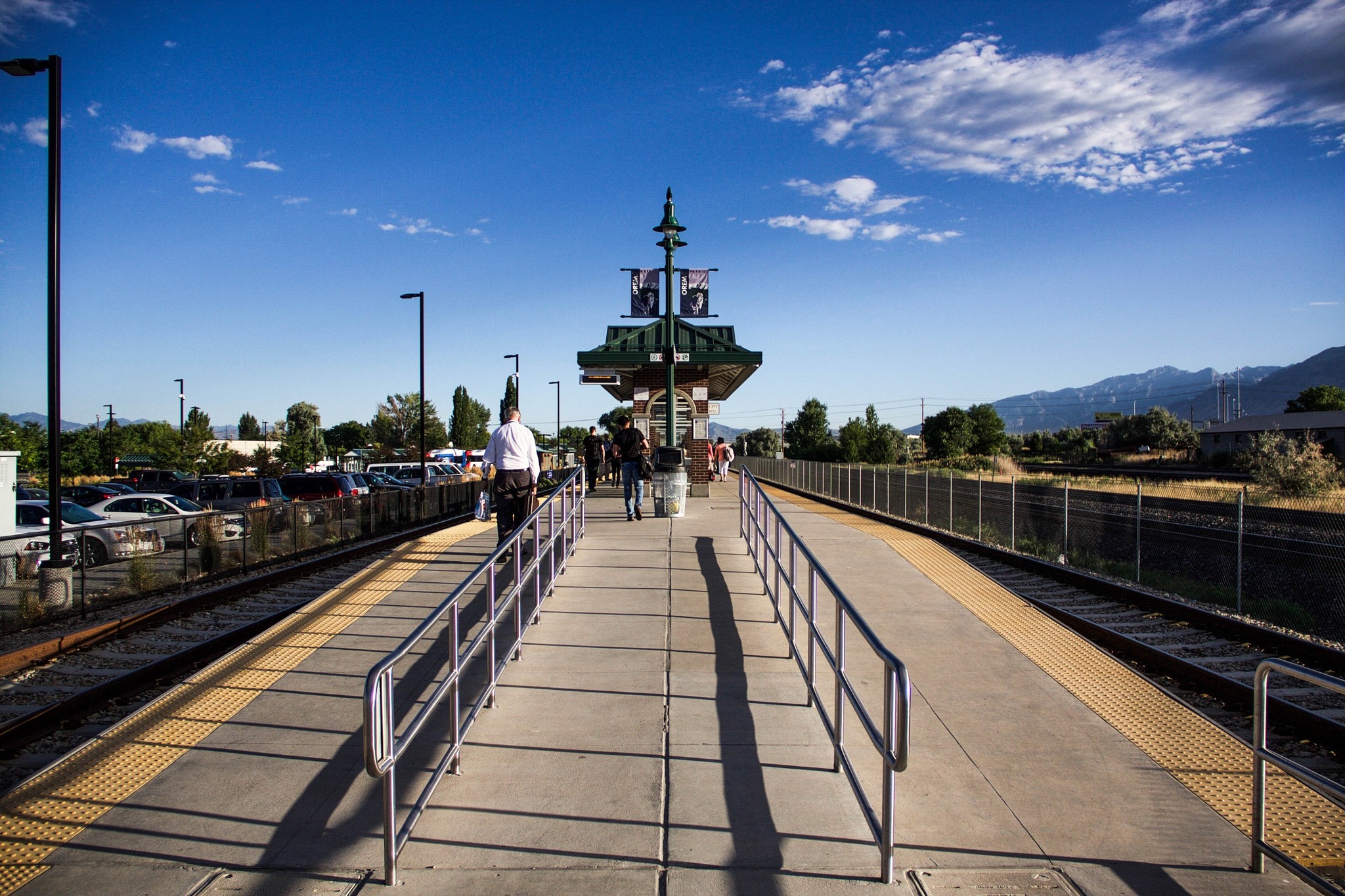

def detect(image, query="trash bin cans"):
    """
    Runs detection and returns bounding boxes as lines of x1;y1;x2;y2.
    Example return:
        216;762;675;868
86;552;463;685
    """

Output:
651;445;688;517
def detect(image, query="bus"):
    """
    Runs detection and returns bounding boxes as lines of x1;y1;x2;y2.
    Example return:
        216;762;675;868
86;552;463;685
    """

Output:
428;448;493;470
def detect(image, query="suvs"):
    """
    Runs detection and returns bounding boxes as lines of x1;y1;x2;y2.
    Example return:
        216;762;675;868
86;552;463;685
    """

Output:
16;469;420;530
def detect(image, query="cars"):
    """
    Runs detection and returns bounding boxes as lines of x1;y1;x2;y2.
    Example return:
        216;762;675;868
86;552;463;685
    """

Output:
86;495;253;549
16;498;166;568
16;526;83;580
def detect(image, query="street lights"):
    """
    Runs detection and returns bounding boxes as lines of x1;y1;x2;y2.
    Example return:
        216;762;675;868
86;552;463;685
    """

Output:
504;353;520;410
2;54;63;563
262;421;269;461
536;433;582;467
175;379;184;453
312;415;319;471
651;186;690;445
400;291;427;487
104;405;117;477
191;407;201;426
549;381;560;466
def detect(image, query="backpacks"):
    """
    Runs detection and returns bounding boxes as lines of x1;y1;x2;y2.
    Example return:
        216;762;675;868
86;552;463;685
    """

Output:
602;441;614;463
586;436;599;459
724;444;735;462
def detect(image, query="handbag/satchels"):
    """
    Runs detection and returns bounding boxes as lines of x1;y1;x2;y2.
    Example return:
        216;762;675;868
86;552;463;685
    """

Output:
639;455;655;479
526;494;539;530
475;489;492;521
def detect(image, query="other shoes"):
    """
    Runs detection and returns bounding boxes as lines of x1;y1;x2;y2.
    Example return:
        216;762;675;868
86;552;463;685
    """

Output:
496;552;507;563
590;488;597;491
512;549;527;556
605;479;612;481
598;479;603;481
616;486;620;488
612;480;615;487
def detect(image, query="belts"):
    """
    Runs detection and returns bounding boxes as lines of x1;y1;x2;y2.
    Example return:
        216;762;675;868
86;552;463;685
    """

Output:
497;468;530;472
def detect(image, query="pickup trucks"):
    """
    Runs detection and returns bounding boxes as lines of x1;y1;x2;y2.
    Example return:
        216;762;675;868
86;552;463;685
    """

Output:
393;466;472;486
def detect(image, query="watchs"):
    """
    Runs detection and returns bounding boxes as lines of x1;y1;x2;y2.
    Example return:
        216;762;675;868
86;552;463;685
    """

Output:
481;476;488;481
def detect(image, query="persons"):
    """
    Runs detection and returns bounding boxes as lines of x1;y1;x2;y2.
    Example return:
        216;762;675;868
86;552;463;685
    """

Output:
481;406;540;562
609;441;622;488
582;426;605;492
712;437;730;482
598;432;613;482
612;415;650;522
708;439;717;481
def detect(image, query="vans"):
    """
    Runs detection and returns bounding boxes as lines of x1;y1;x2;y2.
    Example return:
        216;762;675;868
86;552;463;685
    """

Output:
366;461;481;481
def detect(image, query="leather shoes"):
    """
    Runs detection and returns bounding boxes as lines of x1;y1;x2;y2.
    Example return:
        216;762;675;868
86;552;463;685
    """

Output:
634;505;642;521
627;516;634;521
